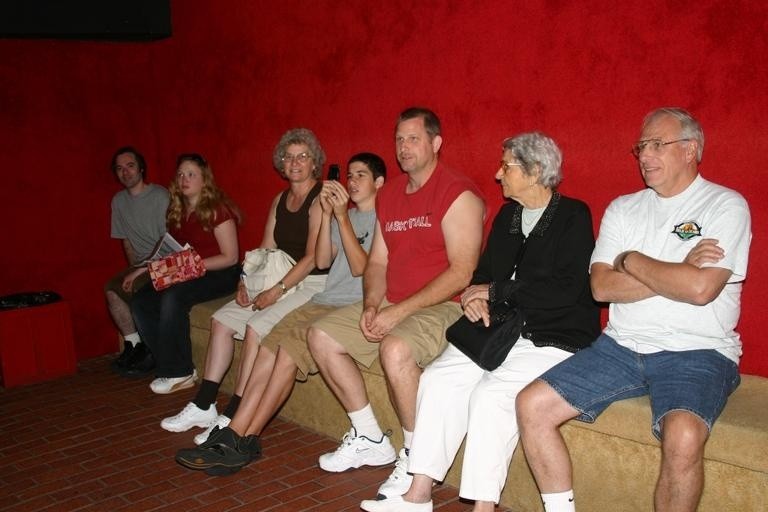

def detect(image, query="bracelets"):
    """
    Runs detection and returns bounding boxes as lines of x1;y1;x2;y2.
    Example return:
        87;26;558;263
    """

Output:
277;281;287;294
620;250;636;270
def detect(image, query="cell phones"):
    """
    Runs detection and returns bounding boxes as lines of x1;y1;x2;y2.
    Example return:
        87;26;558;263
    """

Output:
329;164;339;181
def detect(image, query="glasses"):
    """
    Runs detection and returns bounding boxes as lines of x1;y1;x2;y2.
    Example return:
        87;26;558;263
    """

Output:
281;153;310;162
631;139;690;158
500;161;523;172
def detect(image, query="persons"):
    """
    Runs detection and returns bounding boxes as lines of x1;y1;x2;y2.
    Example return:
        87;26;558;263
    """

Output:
121;151;246;395
511;105;752;511
173;150;386;475
159;124;325;447
102;143;172;372
356;132;603;511
305;107;487;501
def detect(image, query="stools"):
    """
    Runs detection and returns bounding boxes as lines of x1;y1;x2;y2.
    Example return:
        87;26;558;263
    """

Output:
0;292;77;389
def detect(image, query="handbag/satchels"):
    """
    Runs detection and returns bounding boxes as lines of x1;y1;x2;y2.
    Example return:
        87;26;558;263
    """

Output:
242;248;303;308
147;247;206;290
446;303;525;373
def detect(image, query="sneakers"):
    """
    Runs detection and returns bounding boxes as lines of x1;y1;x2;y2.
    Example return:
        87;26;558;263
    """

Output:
161;403;259;477
117;341;198;394
319;427;434;512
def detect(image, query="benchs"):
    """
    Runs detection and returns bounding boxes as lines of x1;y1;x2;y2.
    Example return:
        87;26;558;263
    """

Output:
117;287;767;512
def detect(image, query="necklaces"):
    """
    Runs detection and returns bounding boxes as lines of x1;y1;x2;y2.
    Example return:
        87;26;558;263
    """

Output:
522;200;548;227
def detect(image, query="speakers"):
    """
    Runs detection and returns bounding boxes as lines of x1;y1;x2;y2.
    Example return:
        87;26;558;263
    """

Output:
0;0;171;41
